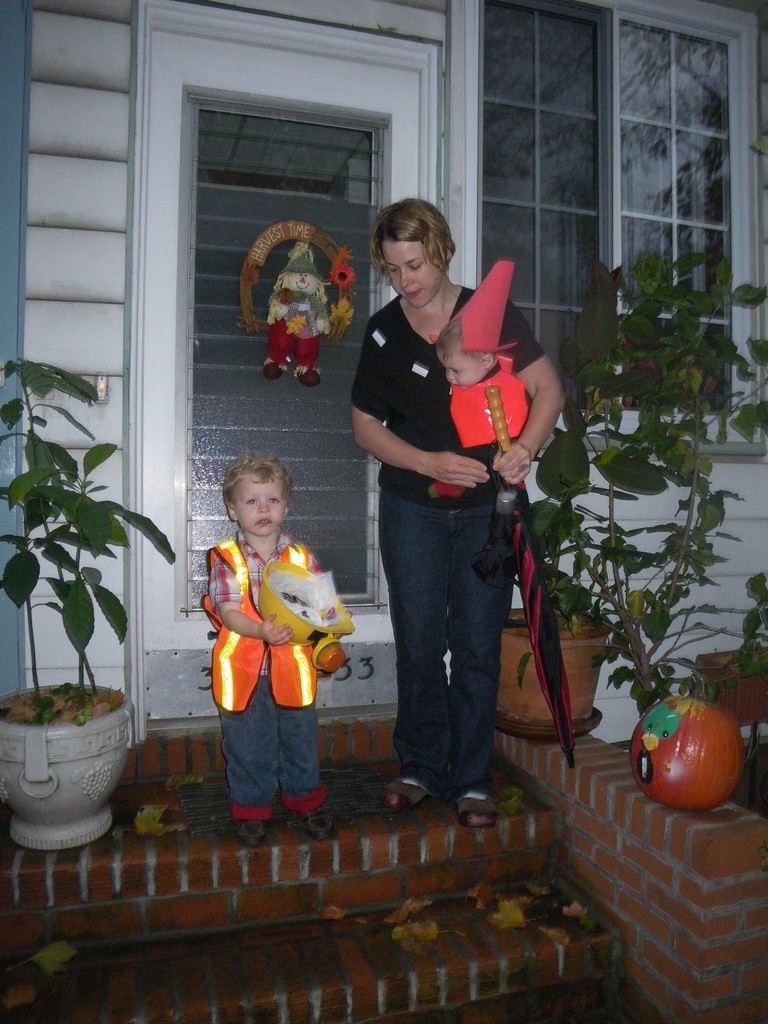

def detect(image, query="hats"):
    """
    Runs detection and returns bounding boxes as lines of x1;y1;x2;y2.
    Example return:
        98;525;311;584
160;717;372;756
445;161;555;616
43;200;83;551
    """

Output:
429;260;518;353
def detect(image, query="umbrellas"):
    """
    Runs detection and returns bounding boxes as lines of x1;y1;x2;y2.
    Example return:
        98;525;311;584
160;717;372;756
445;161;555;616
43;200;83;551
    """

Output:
485;385;577;770
471;484;516;584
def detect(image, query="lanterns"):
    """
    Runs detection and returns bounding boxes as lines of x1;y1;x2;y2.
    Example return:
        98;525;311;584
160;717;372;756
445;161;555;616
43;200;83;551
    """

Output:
628;694;743;812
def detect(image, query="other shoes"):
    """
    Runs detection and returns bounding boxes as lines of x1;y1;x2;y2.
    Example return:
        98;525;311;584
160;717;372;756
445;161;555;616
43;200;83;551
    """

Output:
236;819;270;846
457;794;499;829
377;776;427;818
294;805;335;840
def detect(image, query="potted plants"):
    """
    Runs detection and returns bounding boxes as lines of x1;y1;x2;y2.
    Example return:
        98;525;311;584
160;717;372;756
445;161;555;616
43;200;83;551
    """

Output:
494;259;669;739
0;358;177;850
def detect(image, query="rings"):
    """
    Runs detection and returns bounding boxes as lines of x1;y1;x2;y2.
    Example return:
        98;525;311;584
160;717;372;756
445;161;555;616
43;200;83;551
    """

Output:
519;464;527;471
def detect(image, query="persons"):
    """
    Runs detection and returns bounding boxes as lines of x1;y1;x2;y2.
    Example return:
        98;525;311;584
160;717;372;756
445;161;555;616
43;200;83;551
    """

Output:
207;455;352;847
351;199;566;826
427;315;530;497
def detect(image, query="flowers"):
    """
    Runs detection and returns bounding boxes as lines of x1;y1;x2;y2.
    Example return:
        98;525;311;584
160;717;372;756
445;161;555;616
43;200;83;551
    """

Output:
328;261;355;290
242;263;259;286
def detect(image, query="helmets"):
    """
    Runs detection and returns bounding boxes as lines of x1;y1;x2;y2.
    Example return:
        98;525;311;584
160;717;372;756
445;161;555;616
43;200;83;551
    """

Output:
258;561;355;646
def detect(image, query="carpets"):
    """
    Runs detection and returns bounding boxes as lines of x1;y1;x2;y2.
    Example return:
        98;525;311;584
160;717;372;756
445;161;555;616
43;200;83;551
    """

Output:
174;762;402;839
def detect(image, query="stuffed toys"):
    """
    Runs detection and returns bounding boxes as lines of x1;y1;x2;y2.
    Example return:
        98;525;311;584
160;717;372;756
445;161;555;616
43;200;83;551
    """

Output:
263;245;330;387
